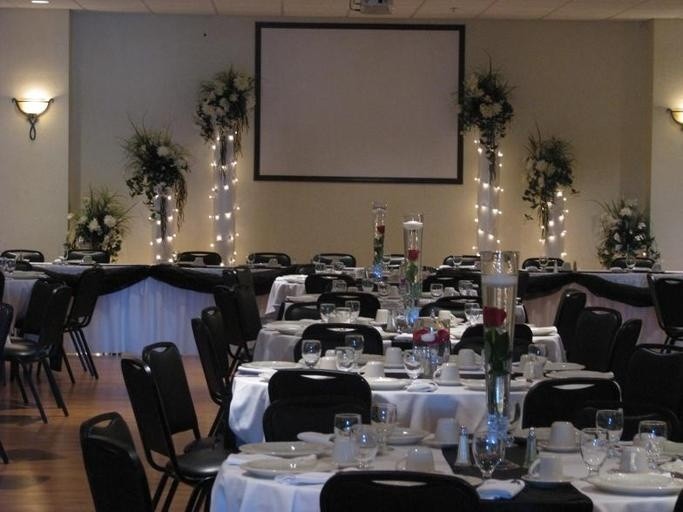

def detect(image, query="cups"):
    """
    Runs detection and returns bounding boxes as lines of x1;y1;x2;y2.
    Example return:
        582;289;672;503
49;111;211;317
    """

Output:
622;445;650;472
435;417;460;443
547;422;586;447
395;448;435;471
527;453;566;480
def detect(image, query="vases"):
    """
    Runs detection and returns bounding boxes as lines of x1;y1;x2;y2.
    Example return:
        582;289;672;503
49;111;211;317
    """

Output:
534;193;567;260
465;135;505;256
400;214;426;303
367;200;391;265
200;117;241;267
142;183;177;267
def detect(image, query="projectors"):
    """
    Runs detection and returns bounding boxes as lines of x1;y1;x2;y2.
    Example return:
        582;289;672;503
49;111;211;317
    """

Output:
359;0;392;15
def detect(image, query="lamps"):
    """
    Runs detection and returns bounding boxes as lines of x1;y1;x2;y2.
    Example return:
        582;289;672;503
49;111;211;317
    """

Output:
664;106;683;136
7;87;56;140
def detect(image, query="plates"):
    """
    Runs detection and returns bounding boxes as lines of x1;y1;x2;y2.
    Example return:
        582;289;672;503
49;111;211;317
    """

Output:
540;441;581;455
547;362;581;371
583;469;683;495
241;356;300;371
542;371;614;379
422;433;459;445
383;425;429;444
241;456;316;477
14;271;44;278
238;440;325;457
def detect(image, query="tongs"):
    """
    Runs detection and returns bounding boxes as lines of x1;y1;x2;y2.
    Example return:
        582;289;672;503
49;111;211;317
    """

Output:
626;256;637;272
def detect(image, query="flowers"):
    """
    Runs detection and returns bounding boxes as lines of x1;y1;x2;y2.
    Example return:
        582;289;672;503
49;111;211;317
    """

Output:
444;43;517;183
60;184;125;259
110;105;192;240
586;189;664;270
513;116;579;248
190;62;258;182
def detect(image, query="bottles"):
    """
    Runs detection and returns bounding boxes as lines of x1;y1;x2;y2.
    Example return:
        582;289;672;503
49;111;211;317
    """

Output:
553;258;578;272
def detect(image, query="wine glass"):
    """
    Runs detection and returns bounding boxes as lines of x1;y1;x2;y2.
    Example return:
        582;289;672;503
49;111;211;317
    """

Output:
273;254;481;336
330;403;398;471
540;255;548;272
637;420;670;471
578;408;623;484
300;334;547;390
